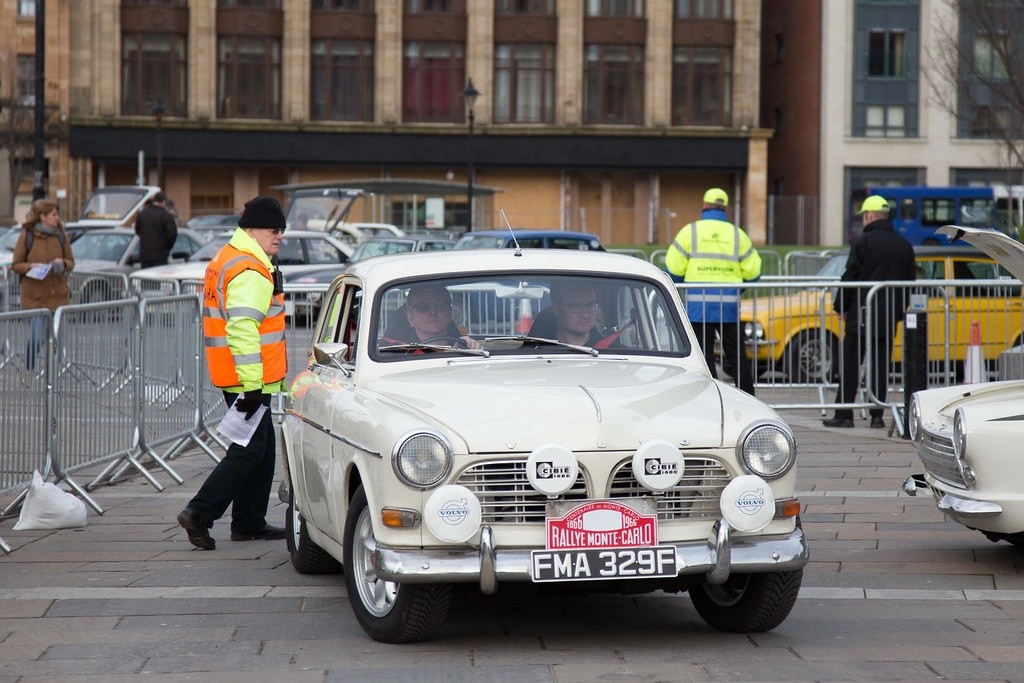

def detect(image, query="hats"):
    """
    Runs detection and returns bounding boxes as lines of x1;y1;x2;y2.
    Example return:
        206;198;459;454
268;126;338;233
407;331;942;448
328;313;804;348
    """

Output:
856;195;890;214
238;195;286;230
703;188;729;207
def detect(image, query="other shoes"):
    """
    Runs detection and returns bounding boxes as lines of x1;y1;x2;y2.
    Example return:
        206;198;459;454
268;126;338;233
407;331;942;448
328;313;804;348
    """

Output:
871;416;886;429
823;413;854;428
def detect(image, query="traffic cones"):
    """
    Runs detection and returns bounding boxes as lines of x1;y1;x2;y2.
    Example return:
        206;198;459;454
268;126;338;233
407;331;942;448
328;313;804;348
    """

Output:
964;321;988;382
516;281;534;335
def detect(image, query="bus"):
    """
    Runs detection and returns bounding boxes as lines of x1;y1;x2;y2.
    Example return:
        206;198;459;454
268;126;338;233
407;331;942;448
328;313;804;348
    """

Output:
847;184;1024;245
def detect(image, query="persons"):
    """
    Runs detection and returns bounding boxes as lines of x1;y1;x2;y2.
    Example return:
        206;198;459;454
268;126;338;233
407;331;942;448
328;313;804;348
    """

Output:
823;196;917;427
134;194;183;289
290;213;311;248
381;285;483;355
550;281;627;348
666;189;763;397
178;198;289;550
13;200;74;388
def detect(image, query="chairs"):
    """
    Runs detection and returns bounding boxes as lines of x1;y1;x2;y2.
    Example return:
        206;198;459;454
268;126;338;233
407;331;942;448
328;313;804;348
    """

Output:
376;302;463;351
521;308;605;347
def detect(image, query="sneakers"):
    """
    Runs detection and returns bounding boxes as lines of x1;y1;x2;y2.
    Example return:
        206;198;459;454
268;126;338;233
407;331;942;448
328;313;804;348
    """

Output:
231;521;288;541
177;508;215;550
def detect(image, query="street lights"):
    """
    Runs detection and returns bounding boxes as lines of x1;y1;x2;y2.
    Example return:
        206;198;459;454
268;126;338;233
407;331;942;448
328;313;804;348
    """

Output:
150;96;166;192
458;78;483;232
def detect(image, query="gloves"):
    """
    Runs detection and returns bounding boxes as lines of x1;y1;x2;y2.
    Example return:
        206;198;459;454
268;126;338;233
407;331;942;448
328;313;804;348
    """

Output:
49;258;65;276
234;389;263;421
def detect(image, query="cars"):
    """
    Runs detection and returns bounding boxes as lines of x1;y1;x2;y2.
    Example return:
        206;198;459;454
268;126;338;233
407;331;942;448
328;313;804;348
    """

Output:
904;225;1024;549
279;247;809;644
0;178;608;338
714;245;1024;383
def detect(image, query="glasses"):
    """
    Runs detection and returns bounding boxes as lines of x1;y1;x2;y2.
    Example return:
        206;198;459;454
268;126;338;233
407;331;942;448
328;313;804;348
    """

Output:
556;300;599;313
260;228;285;236
409;301;451;312
